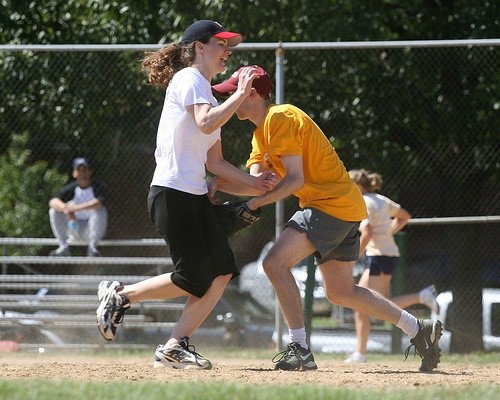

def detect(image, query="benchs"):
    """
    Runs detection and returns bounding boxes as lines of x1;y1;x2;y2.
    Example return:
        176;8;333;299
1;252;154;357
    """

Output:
0;237;220;354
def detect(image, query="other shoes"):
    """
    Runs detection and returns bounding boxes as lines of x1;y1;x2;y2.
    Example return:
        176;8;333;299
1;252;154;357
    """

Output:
88;247;100;257
51;248;71;256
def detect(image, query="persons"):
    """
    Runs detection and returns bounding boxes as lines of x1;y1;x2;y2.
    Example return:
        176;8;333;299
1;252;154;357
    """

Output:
343;168;441;362
47;158;109;256
96;20;279;370
207;66;444;371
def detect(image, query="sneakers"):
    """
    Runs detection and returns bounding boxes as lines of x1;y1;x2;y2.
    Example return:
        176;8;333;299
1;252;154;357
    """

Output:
404;320;443;372
273;343;317;370
96;281;130;341
423;284;439;315
344;355;366;362
154;337;212;369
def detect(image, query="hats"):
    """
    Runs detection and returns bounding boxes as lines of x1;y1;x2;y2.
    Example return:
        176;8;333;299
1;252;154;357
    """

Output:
212;66;272;98
183;20;242;47
74;158;88;168
